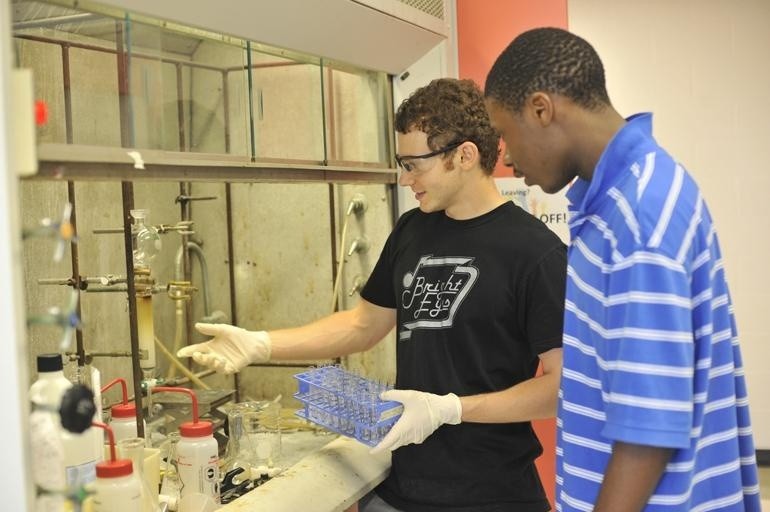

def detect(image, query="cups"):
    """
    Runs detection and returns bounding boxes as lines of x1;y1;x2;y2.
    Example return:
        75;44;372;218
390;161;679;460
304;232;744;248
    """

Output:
151;386;222;511
224;400;283;469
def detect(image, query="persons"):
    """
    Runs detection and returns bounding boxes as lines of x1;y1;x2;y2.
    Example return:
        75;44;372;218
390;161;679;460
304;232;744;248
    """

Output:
484;26;761;512
176;76;569;510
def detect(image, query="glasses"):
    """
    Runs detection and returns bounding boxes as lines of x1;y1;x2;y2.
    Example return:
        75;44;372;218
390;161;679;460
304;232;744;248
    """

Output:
394;142;460;176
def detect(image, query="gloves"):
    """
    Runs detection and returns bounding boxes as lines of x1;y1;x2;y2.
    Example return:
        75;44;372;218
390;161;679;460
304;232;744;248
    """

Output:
370;390;462;454
176;322;271;376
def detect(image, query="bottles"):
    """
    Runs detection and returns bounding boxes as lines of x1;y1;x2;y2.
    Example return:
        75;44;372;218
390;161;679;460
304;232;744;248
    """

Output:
308;361;398;446
91;421;143;511
28;352;76;494
101;377;147;444
